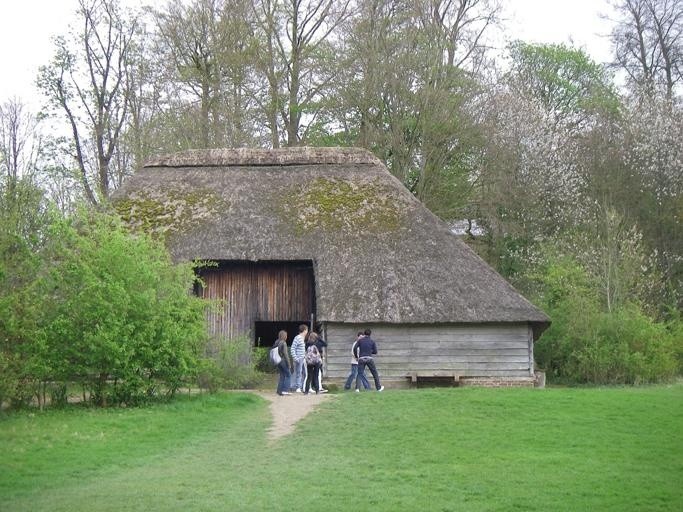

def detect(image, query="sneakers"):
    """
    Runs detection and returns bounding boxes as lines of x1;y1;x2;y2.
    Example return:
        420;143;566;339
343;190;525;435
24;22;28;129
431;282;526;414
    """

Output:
281;388;329;395
343;386;385;393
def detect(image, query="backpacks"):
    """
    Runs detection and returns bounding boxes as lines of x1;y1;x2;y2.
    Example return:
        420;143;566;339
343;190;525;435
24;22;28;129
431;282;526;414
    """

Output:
304;345;323;367
269;346;282;368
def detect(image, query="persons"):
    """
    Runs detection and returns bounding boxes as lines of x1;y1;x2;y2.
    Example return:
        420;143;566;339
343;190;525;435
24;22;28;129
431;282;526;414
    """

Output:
353;329;384;393
273;324;329;396
343;332;373;391
